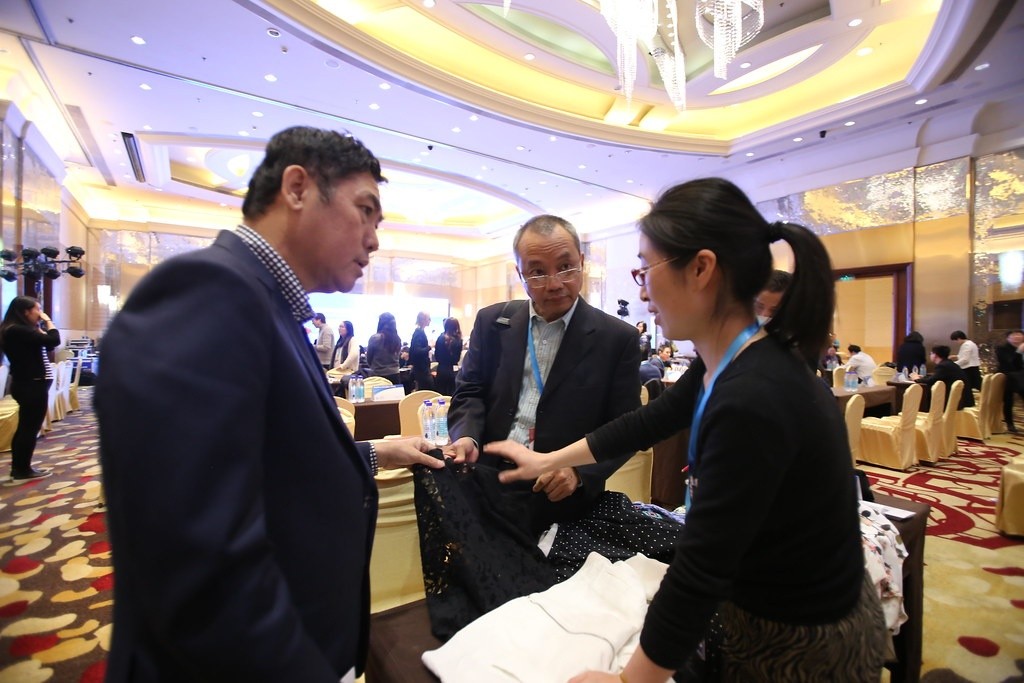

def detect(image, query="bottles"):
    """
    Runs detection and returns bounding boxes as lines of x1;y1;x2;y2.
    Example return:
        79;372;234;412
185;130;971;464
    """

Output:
422;399;448;446
920;365;927;376
843;371;858;391
902;365;909;378
826;357;837;369
913;365;919;374
348;375;365;403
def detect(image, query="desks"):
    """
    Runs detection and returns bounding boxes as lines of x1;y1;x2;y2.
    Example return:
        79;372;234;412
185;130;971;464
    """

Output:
830;384;897;416
367;493;930;683
352;399;402;442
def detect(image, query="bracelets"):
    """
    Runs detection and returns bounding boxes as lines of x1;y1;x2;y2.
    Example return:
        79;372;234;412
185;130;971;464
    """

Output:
44;319;51;323
620;673;627;683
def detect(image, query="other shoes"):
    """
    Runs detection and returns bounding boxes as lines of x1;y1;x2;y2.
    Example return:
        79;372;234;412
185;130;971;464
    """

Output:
11;468;47;477
14;472;50;479
1008;425;1020;433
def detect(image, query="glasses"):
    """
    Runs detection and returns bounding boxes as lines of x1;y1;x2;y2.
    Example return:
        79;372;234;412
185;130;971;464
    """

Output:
520;261;582;289
632;256;680;286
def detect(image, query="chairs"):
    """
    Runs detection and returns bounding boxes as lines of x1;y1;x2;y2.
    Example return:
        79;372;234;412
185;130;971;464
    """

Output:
0;350;82;452
952;374;993;446
321;368;453;442
858;383;923;471
973;373;1005;433
899;381;964;456
833;367;845;388
881;381;947;464
847;395;865;465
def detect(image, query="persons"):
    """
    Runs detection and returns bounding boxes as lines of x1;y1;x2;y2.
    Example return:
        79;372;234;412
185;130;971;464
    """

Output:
311;313;336;369
434;317;462;396
896;331;926;374
442;215;641;501
754;269;792;320
948;330;981;388
824;334;877;384
329;321;360;398
995;329;1024;433
366;312;401;385
485;178;886;683
410;312;435;391
909;345;975;411
399;342;412;368
359;345;364;354
0;295;61;479
635;321;677;399
93;126;443;683
